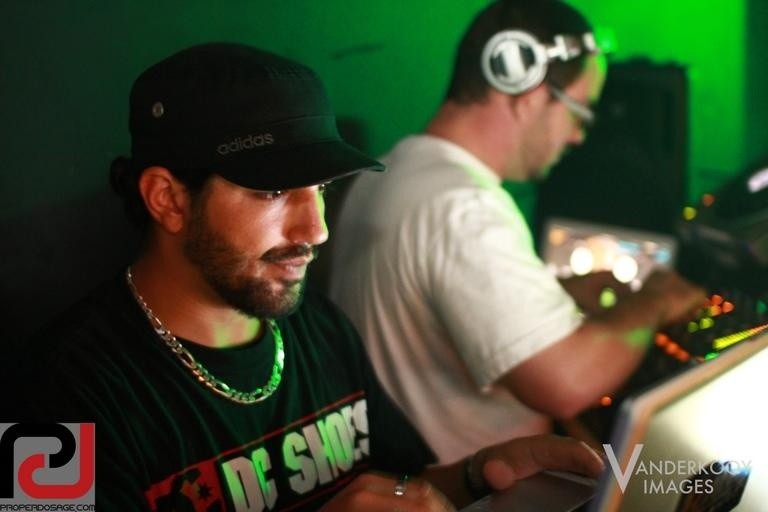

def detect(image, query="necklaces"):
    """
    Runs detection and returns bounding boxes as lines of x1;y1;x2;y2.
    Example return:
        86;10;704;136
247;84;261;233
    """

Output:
127;269;287;405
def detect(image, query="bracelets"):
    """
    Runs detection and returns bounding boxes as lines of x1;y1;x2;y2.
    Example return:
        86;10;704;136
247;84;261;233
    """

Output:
461;456;485;500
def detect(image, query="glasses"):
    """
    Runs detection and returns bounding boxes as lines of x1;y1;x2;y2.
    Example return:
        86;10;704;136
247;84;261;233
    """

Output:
545;85;601;131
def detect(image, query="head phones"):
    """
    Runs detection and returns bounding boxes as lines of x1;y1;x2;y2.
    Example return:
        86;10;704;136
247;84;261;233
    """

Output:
479;24;618;99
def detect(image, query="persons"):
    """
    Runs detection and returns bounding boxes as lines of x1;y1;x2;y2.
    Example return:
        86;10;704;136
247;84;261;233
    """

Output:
0;43;614;511
323;0;708;467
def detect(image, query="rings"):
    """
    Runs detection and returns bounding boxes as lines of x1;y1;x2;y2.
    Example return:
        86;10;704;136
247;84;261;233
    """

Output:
394;470;409;496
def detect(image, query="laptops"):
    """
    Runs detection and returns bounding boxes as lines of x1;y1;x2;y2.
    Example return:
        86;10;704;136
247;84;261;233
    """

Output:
458;328;767;512
540;216;677;306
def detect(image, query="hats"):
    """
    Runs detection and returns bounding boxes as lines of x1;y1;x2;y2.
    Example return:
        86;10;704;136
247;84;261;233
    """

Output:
128;43;388;191
